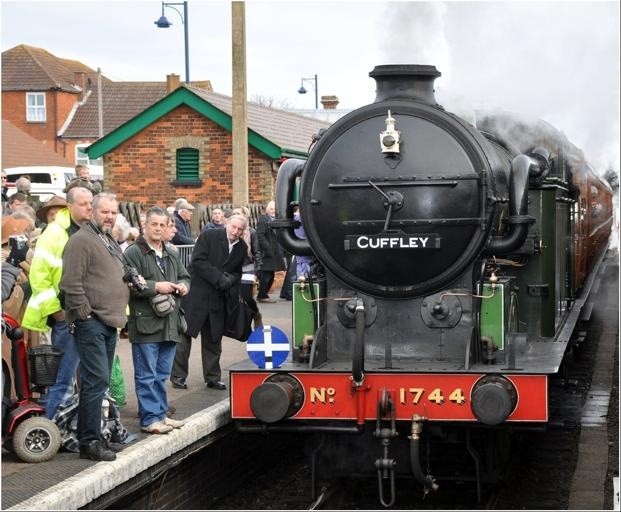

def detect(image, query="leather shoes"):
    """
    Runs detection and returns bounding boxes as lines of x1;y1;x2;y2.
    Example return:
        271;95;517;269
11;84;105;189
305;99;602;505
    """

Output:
208;381;226;389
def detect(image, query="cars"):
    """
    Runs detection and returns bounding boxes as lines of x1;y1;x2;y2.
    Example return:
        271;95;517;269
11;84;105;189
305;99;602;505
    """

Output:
3;188;65;203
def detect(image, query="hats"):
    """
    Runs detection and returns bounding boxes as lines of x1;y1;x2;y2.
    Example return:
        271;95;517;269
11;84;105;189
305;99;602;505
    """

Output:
36;197;67;223
2;217;30;245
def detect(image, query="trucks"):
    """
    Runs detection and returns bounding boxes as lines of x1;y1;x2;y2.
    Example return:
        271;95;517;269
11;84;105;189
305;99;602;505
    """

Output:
3;165;102;194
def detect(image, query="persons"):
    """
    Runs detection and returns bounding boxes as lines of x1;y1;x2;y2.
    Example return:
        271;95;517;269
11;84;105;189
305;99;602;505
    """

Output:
169;198;251;252
168;214;250;392
56;192;149;463
22;186;94;425
254;201;309;302
1;163;198;250
124;205;193;436
0;231;32;431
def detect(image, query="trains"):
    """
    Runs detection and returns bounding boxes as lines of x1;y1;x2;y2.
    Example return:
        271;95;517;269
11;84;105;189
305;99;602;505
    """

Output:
227;64;615;510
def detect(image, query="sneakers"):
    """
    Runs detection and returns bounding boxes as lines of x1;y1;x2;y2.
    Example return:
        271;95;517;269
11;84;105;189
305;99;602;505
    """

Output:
171;375;187;390
142;418;184;432
79;439;124;461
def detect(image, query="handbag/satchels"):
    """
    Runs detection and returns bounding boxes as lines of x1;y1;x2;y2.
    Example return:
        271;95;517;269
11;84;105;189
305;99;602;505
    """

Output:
149;295;175;319
221;299;253;340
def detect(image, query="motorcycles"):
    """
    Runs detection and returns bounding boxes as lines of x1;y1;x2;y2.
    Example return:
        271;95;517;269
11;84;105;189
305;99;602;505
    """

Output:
1;308;60;462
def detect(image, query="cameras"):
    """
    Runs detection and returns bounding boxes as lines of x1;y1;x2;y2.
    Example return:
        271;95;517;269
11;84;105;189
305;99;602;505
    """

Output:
122;264;149;292
6;234;28;265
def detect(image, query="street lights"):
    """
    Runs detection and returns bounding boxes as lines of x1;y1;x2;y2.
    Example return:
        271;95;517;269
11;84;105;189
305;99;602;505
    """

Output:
73;66;105;142
297;74;318;110
154;2;190;84
